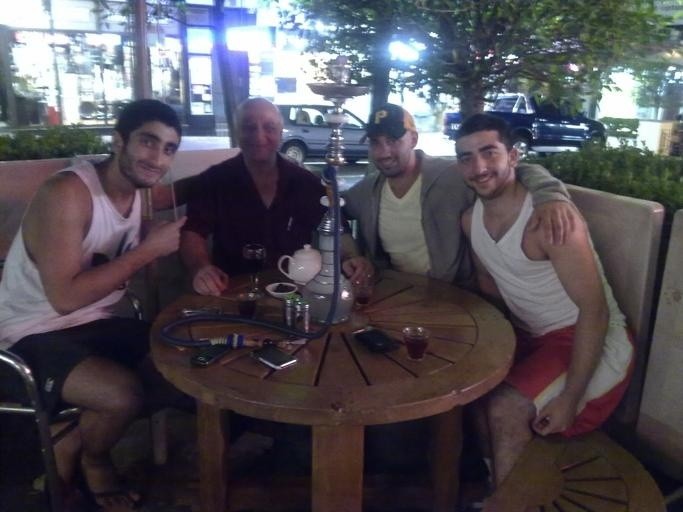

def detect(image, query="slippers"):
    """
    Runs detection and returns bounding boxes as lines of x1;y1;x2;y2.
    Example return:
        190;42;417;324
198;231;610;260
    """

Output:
72;458;141;511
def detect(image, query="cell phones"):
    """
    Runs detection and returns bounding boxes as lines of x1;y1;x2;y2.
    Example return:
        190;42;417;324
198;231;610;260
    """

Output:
182;303;223;317
254;344;297;370
190;343;232;367
263;270;289;288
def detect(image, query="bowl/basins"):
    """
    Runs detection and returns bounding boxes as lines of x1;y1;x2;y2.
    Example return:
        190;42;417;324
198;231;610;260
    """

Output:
265;283;298;298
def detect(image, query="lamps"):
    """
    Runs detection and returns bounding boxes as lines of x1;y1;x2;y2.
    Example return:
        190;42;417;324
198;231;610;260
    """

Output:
306;55;371;166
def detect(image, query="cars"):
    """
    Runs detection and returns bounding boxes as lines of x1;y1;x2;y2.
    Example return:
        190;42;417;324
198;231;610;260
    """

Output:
276;98;373;166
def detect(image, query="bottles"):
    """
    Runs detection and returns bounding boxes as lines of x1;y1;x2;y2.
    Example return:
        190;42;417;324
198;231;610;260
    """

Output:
284;294;311;335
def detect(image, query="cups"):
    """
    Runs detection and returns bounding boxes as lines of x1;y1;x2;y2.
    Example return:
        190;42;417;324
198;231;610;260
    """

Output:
234;294;260;318
351;272;375;312
403;327;432;362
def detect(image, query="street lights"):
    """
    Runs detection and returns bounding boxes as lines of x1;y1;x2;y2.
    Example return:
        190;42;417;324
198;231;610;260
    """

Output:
42;0;63;125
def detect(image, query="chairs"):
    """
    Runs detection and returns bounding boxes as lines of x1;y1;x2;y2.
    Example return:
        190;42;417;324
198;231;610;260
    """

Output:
0;344;88;512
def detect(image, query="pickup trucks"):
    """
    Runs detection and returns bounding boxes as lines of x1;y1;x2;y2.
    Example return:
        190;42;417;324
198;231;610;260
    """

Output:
443;92;607;156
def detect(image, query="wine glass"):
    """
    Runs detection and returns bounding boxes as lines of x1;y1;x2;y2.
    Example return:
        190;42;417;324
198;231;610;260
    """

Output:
244;244;267;299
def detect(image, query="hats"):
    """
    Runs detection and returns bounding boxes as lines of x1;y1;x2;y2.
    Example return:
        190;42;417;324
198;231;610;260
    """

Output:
359;102;417;144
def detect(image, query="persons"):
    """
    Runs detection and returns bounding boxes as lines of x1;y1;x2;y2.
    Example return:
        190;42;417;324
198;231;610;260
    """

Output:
453;117;639;491
0;97;306;510
330;100;581;511
176;94;377;492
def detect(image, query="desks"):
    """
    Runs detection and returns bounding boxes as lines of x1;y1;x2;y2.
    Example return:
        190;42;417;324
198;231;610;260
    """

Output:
150;267;516;512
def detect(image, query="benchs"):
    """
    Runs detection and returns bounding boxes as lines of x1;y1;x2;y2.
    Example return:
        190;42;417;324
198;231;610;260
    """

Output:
482;424;667;511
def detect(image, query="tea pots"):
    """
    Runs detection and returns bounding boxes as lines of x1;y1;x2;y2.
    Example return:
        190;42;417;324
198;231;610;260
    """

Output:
277;243;323;284
